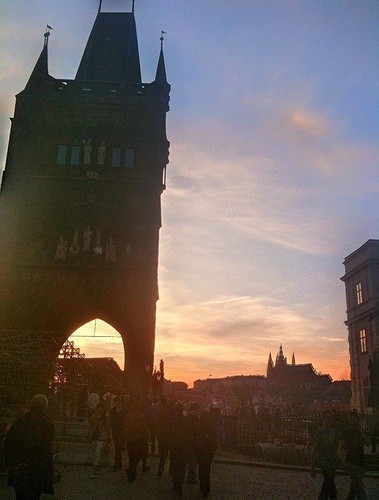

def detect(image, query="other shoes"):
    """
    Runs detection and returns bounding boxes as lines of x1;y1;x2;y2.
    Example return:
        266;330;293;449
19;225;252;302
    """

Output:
142;465;150;472
158;468;163;476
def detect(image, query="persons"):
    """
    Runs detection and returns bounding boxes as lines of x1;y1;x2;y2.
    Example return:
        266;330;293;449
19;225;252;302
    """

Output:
0;395;57;499
79;385;222;500
307;410;373;499
